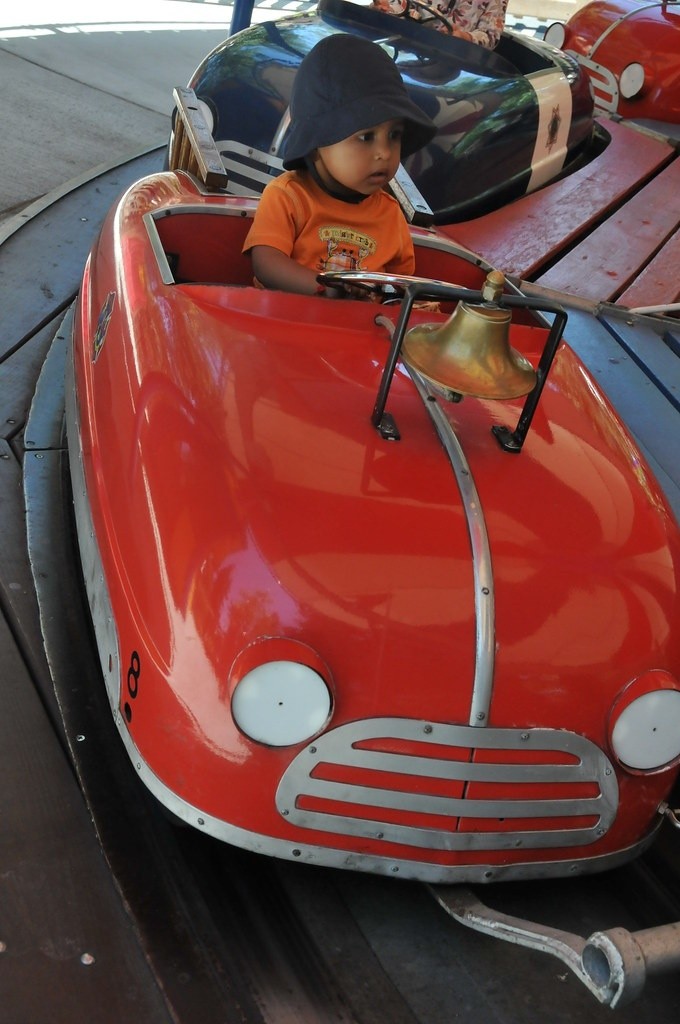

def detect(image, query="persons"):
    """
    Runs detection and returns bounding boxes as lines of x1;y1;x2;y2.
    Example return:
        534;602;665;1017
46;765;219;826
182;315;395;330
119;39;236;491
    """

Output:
241;34;438;308
370;0;509;52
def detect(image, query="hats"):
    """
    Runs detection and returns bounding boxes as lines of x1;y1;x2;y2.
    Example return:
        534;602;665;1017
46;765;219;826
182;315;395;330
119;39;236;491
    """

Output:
283;34;438;170
